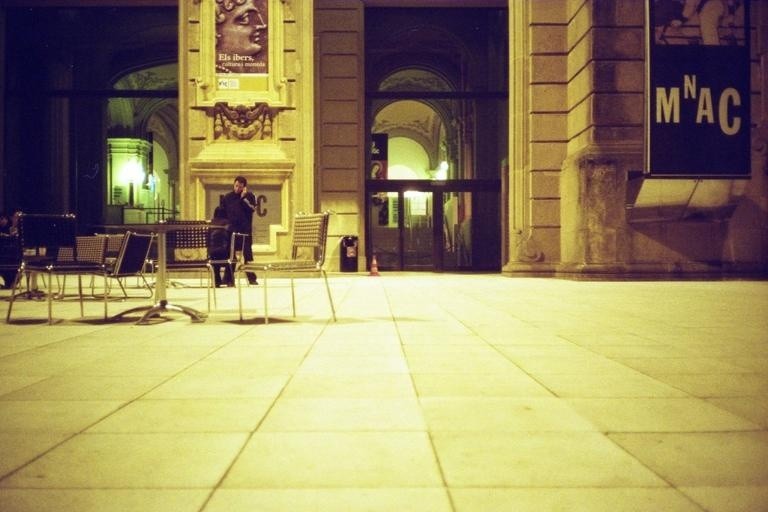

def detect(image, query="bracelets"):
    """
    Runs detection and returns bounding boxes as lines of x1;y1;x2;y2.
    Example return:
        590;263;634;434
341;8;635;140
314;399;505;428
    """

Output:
240;196;247;200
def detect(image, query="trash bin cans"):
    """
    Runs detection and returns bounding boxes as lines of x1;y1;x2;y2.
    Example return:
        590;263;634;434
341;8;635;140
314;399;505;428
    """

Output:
340;235;358;271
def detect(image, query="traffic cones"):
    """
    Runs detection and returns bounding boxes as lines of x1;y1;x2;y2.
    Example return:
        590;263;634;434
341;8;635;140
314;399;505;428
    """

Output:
367;254;382;276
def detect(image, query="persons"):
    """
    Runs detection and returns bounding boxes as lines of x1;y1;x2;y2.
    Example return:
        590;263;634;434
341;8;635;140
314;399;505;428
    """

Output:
671;0;742;46
219;174;259;285
204;203;236;288
0;205;29;289
0;212;11;235
215;0;268;73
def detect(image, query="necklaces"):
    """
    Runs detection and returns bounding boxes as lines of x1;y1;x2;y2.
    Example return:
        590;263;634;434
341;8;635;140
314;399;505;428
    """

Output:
215;65;233;74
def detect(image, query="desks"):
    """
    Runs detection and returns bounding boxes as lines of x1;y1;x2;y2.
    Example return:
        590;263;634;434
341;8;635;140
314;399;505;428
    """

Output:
103;222;226;324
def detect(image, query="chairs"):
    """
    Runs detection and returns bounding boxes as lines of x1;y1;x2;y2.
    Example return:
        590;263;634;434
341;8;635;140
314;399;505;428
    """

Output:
7;213;108;326
237;212;337;325
151;219;219;312
7;232;158;301
200;231;250;287
